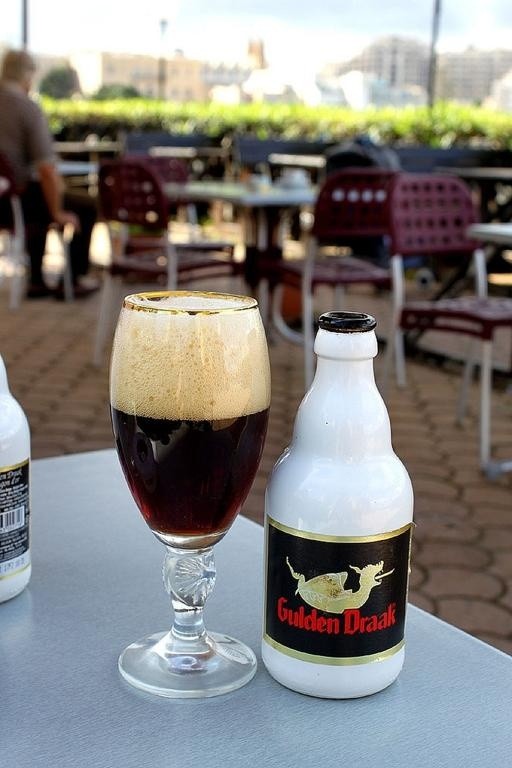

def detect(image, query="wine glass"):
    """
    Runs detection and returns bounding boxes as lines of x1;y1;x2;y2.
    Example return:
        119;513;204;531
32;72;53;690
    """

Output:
104;292;273;703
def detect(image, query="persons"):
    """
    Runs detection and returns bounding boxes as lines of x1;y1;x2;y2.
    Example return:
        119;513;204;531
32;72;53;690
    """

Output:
2;45;106;305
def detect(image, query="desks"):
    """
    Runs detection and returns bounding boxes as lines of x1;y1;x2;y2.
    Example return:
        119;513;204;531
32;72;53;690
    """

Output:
0;447;511;768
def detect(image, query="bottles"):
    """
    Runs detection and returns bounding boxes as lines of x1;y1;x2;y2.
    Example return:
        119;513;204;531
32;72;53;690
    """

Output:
0;342;35;605
259;310;418;705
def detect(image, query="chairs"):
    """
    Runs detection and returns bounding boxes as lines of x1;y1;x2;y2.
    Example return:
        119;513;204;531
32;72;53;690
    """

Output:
0;135;512;481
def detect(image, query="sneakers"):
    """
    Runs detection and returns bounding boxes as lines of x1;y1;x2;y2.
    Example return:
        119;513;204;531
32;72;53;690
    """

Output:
25;274;101;302
